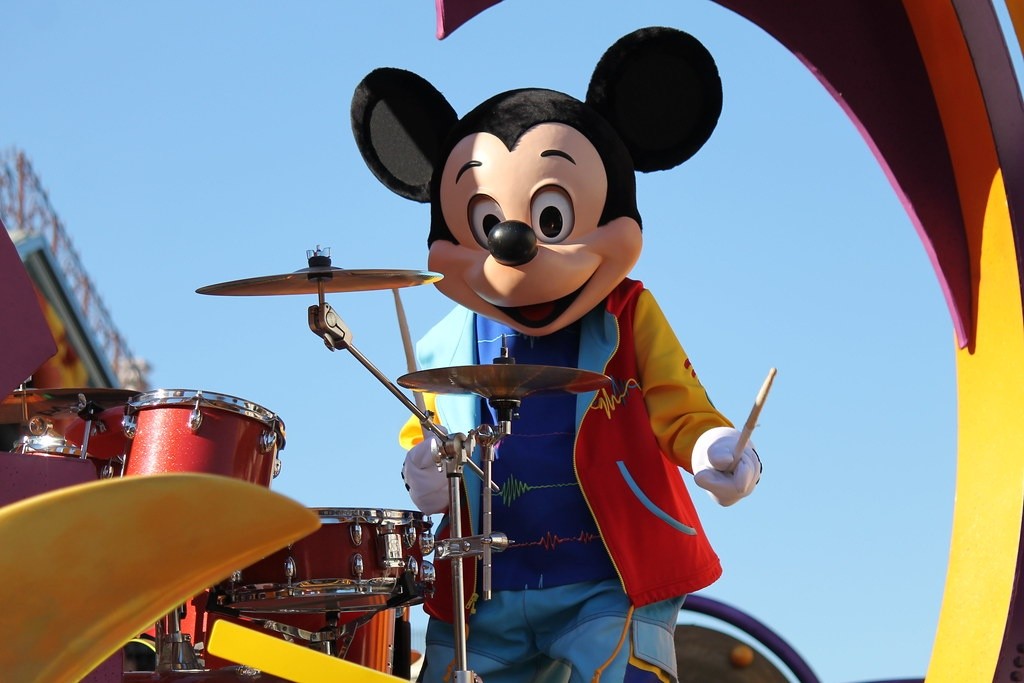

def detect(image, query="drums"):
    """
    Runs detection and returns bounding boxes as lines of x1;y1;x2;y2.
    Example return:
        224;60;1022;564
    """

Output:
14;398;140;465
218;505;426;615
122;386;286;490
145;579;399;683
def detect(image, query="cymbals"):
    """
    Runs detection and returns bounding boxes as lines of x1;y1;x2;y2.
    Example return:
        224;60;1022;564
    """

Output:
395;329;612;400
194;241;446;299
11;385;144;421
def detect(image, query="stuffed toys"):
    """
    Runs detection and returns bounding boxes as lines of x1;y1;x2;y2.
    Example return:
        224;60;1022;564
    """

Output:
350;26;761;683
